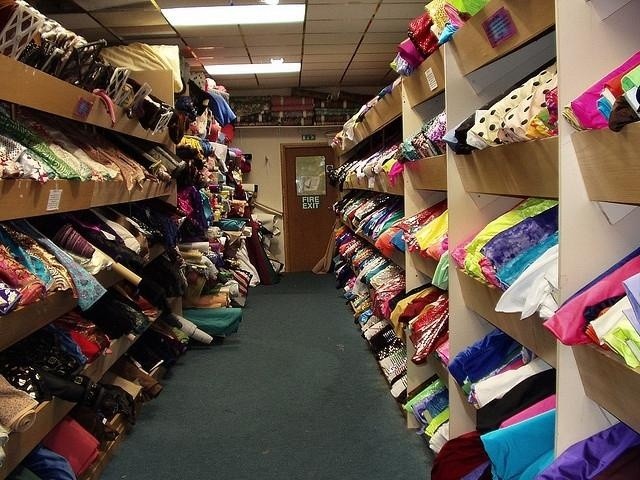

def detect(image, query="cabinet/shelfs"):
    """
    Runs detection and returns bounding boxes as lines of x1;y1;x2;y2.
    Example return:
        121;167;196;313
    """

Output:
0;48;184;479
331;0;640;466
